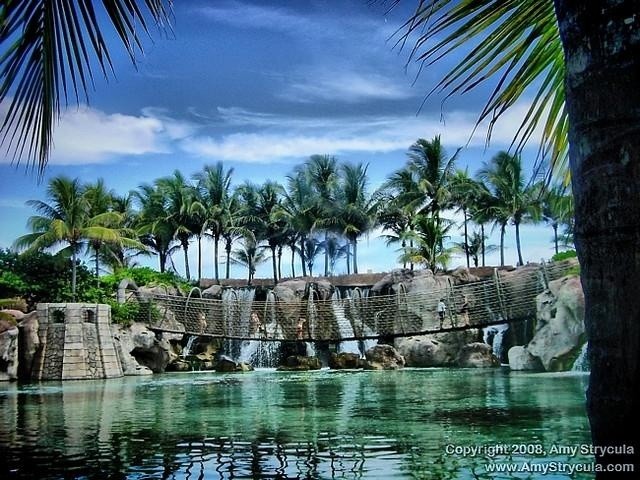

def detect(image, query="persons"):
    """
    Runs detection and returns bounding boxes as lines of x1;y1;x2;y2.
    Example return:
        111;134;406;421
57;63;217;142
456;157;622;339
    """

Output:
458;294;471;326
197;309;206;334
438;298;446;330
296;318;306;339
251;310;259;334
